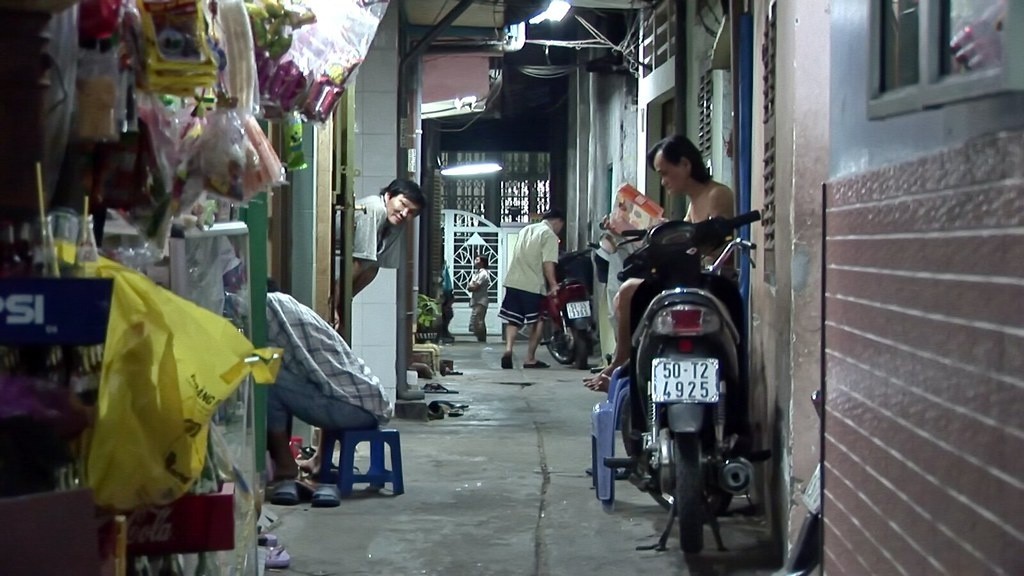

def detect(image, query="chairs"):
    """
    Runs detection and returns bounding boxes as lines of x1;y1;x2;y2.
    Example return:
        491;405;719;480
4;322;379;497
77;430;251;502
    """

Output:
591;365;633;514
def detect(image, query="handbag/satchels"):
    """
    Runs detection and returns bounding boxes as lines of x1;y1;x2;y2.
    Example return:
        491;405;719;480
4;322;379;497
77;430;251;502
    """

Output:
49;240;284;512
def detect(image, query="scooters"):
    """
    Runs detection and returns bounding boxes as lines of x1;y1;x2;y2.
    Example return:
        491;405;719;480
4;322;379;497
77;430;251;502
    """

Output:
543;247;593;370
596;209;763;555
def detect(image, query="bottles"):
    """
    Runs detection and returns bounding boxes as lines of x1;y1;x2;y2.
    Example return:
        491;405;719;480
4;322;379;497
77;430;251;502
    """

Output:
75;215;98;264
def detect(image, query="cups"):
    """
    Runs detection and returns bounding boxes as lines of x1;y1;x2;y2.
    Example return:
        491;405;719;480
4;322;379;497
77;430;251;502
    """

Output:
289;436;302;458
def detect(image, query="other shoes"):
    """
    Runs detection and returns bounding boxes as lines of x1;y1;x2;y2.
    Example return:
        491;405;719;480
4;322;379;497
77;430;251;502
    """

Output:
258;533;290;568
428;401;444;419
437;400;468;416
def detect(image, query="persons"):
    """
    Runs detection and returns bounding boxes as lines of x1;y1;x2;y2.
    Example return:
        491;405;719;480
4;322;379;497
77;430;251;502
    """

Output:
229;271;393;488
501;209;567;368
581;130;733;395
328;177;426;331
466;254;491;342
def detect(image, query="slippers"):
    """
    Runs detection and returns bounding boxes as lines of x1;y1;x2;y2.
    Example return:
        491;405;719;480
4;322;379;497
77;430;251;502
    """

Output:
313;484;341;506
294;442;316;458
523;360;550;368
271;479;297;504
421;383;459;393
501;352;513;369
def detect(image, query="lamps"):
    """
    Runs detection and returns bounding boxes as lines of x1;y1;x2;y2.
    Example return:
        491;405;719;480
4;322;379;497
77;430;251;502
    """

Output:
440;159;506;177
501;0;635;66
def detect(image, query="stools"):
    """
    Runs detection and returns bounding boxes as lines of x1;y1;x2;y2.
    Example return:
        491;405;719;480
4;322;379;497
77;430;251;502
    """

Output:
322;427;403;498
